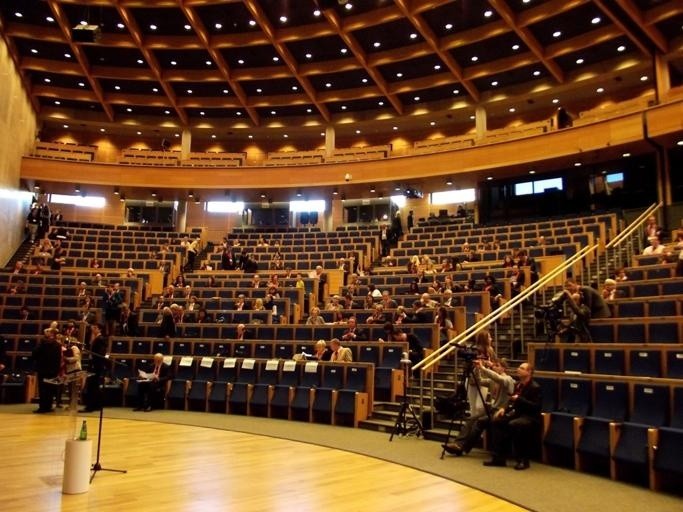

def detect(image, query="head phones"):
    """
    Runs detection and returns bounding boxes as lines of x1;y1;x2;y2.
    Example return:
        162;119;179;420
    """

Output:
579;292;584;304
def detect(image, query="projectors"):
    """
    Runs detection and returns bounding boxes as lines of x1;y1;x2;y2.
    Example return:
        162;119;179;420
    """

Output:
403;189;424;199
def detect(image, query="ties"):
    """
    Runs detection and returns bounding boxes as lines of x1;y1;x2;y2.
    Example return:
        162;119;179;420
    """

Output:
238;334;242;339
155;365;159;375
333;351;339;361
350;328;353;333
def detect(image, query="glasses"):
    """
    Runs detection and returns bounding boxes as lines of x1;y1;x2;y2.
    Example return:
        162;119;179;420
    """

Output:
519;366;526;371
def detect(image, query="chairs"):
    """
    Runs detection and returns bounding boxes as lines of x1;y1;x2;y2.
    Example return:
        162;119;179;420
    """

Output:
0;119;683;500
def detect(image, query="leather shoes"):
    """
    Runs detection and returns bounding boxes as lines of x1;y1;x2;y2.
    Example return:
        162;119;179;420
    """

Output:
78;406;104;413
33;406;63;414
513;461;531;471
133;406;154;412
483;460;507;468
441;443;463;457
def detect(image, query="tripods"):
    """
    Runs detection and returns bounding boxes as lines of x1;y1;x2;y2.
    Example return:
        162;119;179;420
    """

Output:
389;365;424;442
80;348;127;484
440;363;491;460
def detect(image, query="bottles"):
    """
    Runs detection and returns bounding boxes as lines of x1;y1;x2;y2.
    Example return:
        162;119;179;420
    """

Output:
80;420;87;440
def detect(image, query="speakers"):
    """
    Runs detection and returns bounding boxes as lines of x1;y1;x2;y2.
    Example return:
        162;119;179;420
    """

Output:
309;212;318;224
299;212;309;224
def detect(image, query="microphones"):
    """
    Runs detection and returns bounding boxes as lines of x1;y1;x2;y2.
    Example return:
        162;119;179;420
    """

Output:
64;337;86;346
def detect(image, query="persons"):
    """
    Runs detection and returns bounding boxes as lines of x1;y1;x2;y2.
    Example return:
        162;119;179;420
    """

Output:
10;280;24;295
441;331;542;470
380;209;414;256
155;266;306;340
565;269;627;359
644;215;683;256
537;235;550;246
78;274;138;336
91;258;100;268
121;267;136;278
339;317;361;341
481;238;503;249
11;261;27;274
302;340;356;361
21;306;35;320
462;241;472;253
222;236;258;273
456;205;466;217
26;203;67;275
306;250;542;325
133;353;169;411
375;321;424;377
258;238;270;246
273;240;279;246
152;234;214;271
24;319;108;413
271;251;283;270
